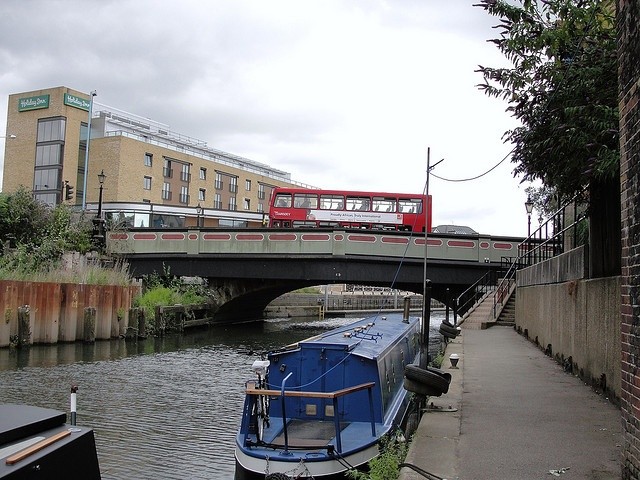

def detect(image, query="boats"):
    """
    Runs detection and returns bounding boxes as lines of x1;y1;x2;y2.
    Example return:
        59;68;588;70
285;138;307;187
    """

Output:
232;298;423;479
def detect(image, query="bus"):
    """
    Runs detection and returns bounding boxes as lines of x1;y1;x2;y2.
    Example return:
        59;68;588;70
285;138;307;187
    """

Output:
267;186;433;233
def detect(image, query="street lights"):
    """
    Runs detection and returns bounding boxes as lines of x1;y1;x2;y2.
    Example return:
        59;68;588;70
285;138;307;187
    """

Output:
92;169;108;239
195;202;202;227
538;215;544;245
524;194;534;266
1;133;17;141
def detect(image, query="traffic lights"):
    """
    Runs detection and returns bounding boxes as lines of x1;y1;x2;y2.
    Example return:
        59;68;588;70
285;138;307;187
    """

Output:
66;185;74;200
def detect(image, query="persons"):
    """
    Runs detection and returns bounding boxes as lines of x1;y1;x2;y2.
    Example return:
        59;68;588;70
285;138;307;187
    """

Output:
359;200;367;210
336;203;342;210
277;199;284;207
